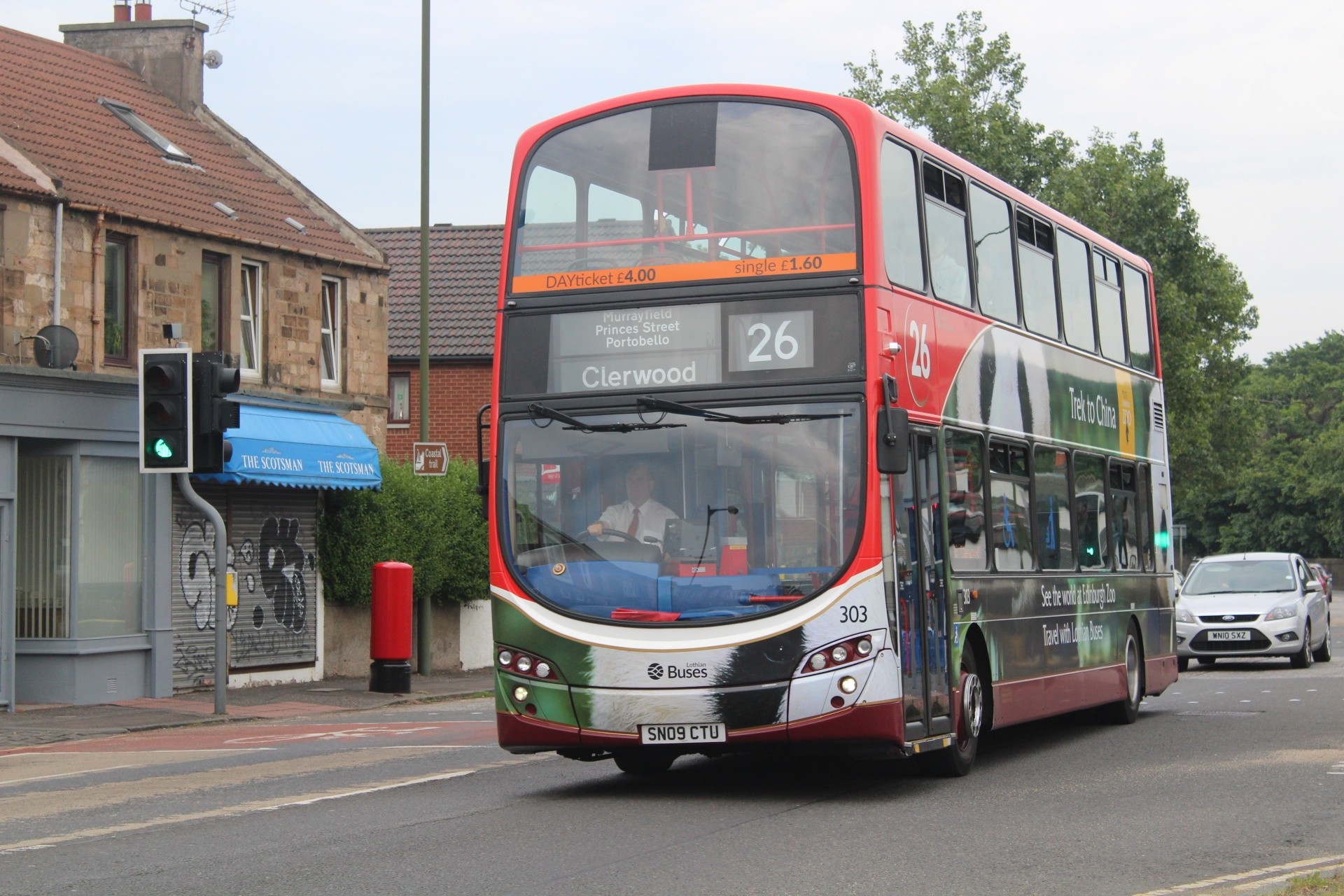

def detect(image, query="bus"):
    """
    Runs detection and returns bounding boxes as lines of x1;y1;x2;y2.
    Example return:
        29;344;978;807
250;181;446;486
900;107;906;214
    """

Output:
476;84;1174;777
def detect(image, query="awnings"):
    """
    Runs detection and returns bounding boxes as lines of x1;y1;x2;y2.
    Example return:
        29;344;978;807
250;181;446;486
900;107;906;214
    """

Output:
184;401;382;492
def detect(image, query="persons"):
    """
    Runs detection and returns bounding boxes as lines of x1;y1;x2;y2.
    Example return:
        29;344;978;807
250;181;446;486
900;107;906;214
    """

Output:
587;465;679;556
718;490;746;538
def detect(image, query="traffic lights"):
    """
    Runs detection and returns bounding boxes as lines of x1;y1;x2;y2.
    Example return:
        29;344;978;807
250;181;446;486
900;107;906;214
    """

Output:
136;348;196;473
197;352;241;474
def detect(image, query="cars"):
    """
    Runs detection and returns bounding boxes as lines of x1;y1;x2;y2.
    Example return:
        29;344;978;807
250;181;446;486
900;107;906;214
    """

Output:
1172;552;1333;667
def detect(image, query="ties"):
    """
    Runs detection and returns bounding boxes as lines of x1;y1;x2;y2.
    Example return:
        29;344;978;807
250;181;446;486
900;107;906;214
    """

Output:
625;508;639;543
